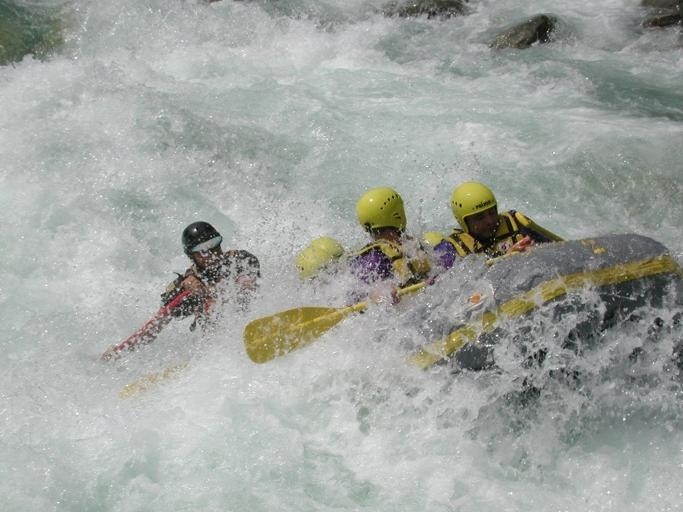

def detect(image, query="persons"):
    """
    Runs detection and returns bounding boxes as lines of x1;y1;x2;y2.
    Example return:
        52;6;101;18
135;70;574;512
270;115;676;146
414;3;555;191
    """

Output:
295;181;566;315
159;220;262;342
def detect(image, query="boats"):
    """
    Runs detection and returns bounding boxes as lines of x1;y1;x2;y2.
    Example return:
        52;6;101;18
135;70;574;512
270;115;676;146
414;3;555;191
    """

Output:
385;228;683;378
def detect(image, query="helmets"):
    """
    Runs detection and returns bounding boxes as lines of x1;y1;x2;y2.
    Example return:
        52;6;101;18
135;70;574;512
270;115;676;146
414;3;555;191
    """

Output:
356;188;406;233
294;248;332;281
181;221;222;253
449;181;498;234
309;236;343;257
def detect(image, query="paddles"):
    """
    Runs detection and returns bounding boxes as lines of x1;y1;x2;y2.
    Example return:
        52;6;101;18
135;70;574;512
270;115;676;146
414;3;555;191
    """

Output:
246;252;505;365
